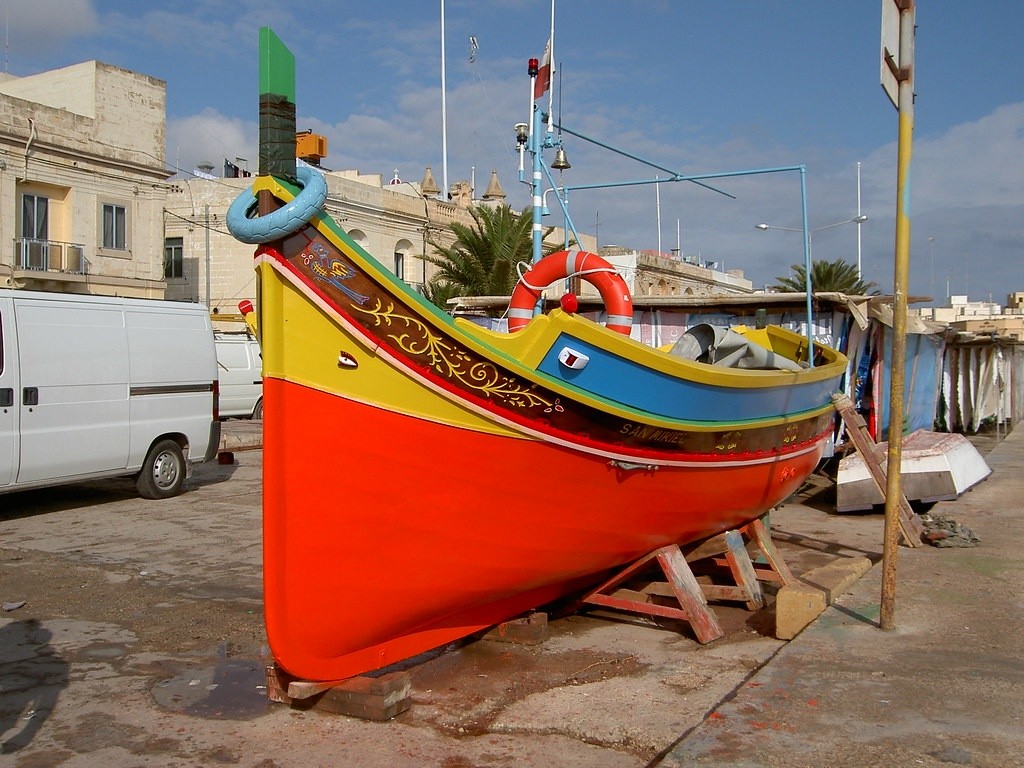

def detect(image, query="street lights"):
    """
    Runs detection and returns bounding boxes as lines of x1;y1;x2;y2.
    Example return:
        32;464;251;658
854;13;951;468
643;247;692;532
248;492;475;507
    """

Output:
755;215;869;273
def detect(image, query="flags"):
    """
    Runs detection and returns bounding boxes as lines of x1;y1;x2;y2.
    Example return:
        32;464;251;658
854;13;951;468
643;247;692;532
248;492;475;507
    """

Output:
535;38;556;99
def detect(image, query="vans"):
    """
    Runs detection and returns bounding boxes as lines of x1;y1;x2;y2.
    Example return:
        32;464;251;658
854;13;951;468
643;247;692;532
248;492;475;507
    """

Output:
0;288;223;500
213;329;263;422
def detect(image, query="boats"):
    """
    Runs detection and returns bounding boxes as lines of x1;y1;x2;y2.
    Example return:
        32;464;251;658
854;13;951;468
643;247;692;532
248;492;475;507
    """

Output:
237;0;851;684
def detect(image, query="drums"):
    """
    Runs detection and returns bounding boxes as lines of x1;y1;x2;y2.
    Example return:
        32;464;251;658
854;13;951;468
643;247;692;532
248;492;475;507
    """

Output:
508;251;633;345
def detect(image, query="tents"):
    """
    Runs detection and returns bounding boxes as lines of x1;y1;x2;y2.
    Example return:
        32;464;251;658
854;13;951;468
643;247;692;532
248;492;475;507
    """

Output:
445;293;1024;459
